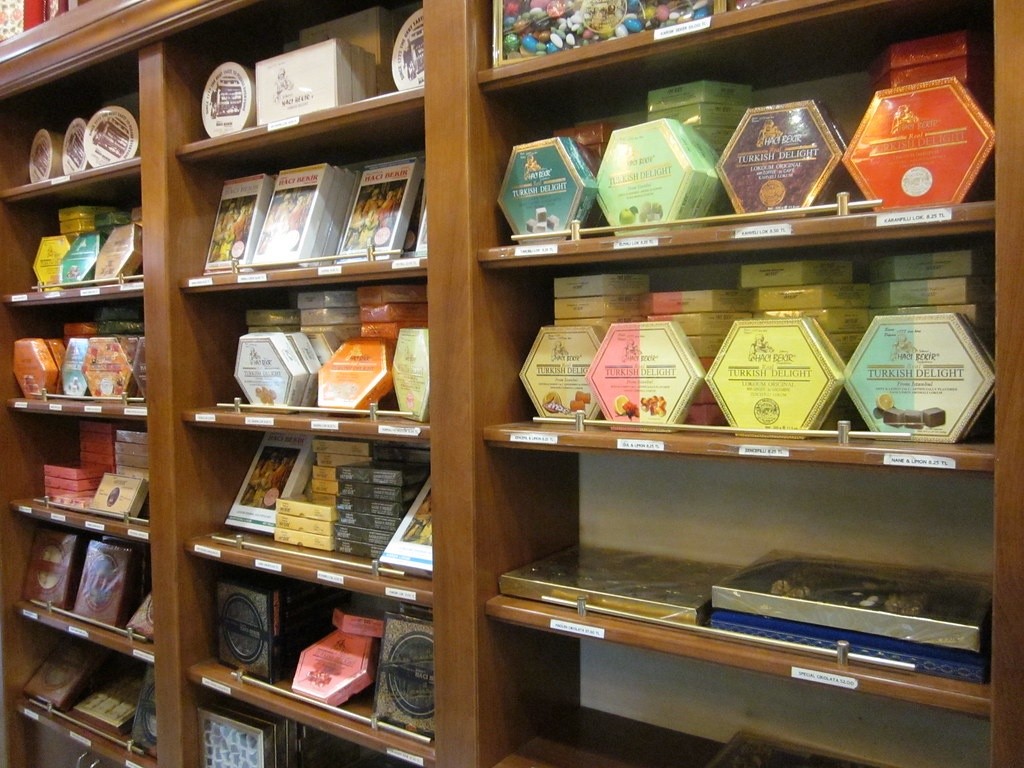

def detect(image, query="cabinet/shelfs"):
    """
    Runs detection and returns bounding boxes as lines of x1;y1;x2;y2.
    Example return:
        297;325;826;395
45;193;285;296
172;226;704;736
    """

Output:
0;0;1024;768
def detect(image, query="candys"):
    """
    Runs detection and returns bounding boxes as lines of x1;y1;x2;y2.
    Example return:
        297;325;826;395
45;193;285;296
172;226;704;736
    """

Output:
504;0;772;57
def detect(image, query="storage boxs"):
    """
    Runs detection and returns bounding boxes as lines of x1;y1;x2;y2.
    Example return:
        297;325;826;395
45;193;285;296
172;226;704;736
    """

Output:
80;441;114;455
22;528;85;610
80;461;115;474
204;156;429;270
43;460;111;480
114;442;147;457
117;465;149;483
22;642;157;759
115;454;148;469
251;5;405;128
44;475;101;491
45;487;97;501
80;451;115;465
90;472;149;518
217;432;433;685
79;430;115;444
80;420;140;433
116;428;148;445
73;538;140;627
126;592;154;641
196;693;360;768
